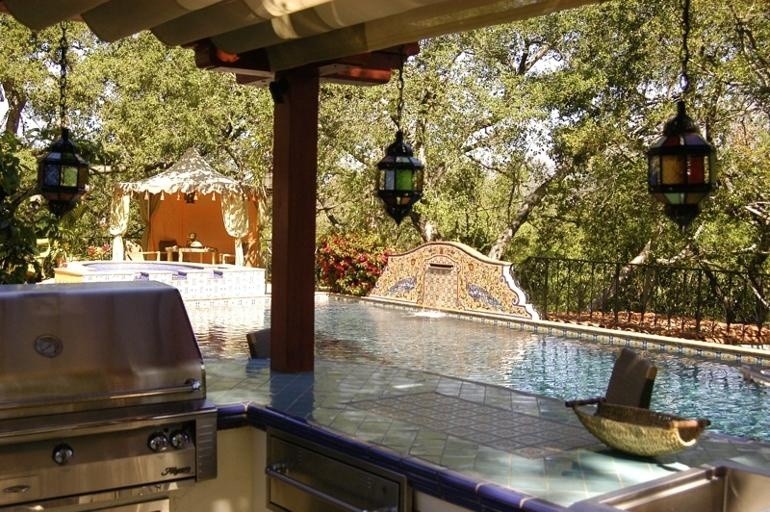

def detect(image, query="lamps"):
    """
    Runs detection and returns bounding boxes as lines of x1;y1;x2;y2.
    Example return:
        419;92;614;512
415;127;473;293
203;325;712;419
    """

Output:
36;18;90;217
375;46;424;225
646;2;719;228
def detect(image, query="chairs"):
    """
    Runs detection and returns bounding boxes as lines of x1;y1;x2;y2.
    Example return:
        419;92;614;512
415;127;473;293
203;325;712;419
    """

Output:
127;242;251;265
566;346;657;420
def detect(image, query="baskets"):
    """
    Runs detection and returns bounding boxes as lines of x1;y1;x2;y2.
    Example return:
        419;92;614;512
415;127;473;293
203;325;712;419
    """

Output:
565;396;711;458
